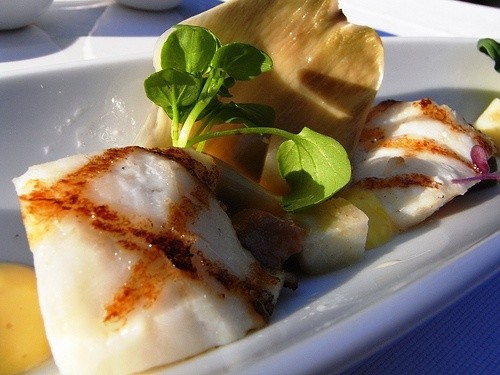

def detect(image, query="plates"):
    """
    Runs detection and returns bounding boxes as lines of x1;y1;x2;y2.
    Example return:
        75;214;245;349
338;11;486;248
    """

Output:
0;39;500;375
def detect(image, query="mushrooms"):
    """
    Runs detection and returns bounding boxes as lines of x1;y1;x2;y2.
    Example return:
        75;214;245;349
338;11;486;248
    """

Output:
151;0;384;193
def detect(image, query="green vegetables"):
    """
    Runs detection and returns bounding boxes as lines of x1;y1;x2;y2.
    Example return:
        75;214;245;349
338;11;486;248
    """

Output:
143;25;352;213
